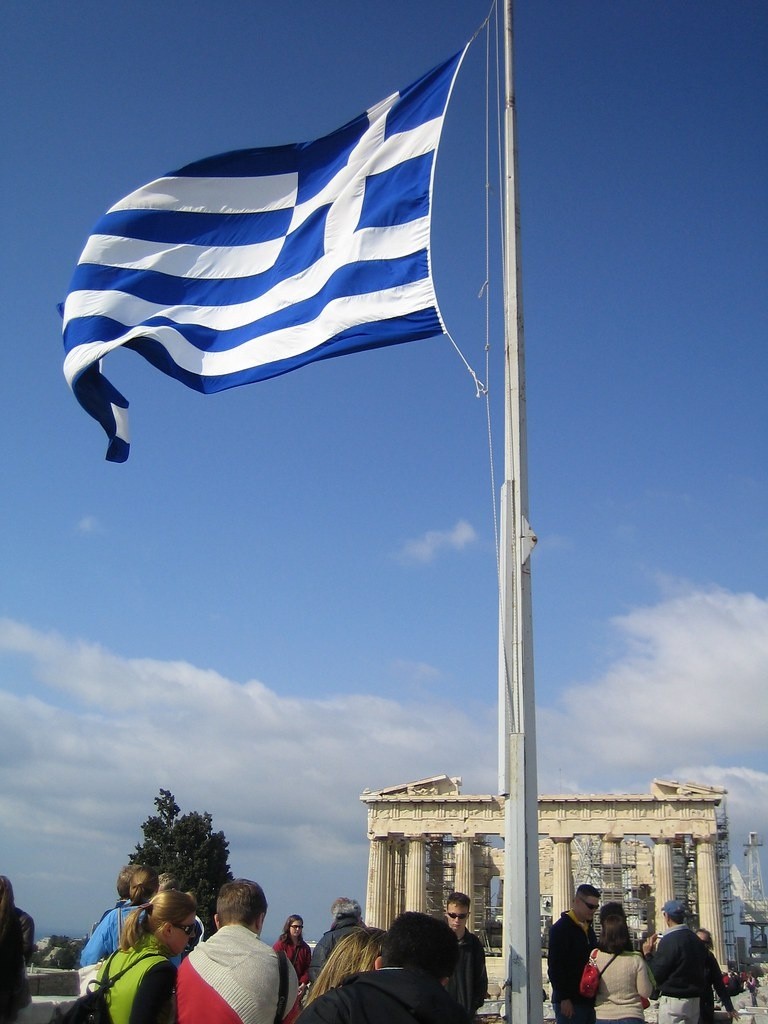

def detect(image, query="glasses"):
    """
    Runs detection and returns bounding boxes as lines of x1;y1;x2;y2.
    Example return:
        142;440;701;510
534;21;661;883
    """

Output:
448;911;468;920
290;924;304;929
170;921;196;936
580;896;599;910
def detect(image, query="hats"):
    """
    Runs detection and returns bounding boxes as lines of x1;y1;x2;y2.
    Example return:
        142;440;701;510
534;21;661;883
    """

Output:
660;901;685;917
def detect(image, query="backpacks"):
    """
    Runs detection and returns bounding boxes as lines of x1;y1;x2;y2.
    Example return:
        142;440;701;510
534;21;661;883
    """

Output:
47;949;159;1024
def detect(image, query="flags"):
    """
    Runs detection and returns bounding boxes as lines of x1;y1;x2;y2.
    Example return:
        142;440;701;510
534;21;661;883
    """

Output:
56;37;483;463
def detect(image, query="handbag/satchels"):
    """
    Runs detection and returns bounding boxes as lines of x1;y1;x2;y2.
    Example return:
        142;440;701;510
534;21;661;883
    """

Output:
580;950;601;999
78;958;107;998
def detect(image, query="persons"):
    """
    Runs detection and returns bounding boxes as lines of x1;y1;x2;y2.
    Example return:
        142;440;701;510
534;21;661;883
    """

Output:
78;864;488;1024
547;884;741;1024
0;875;39;1024
713;967;758;1007
